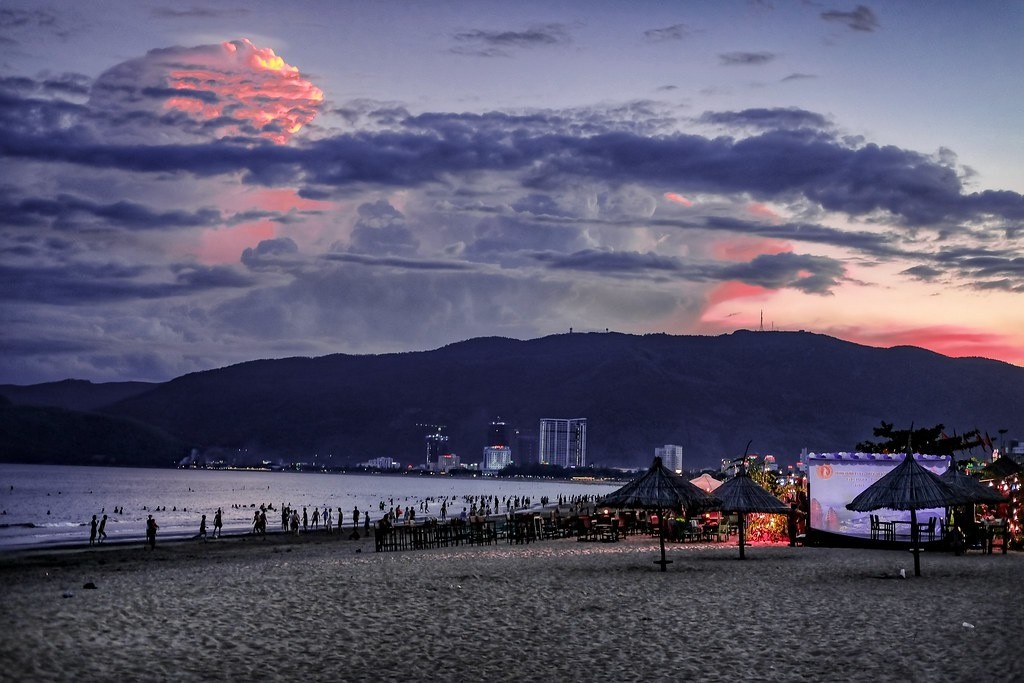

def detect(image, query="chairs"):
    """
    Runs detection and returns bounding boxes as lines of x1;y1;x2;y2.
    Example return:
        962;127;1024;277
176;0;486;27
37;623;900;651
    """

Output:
869;514;893;541
375;510;737;552
918;517;936;542
939;517;956;540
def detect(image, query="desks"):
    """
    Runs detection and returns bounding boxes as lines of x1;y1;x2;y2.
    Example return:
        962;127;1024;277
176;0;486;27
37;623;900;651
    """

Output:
394;523;424;551
891;521;914;542
436;521;454;548
595;524;613;542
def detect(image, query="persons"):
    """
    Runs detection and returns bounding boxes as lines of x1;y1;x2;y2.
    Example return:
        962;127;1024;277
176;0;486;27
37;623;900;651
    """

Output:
81;492;616;558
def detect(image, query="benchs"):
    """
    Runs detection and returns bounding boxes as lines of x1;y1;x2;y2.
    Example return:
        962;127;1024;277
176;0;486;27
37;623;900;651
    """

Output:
796;534;806;547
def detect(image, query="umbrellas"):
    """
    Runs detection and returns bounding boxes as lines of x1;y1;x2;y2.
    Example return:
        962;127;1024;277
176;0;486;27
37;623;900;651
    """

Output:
931;462;1010;551
702;467;794;560
843;443;969;577
599;456;725;572
685;470;725;494
